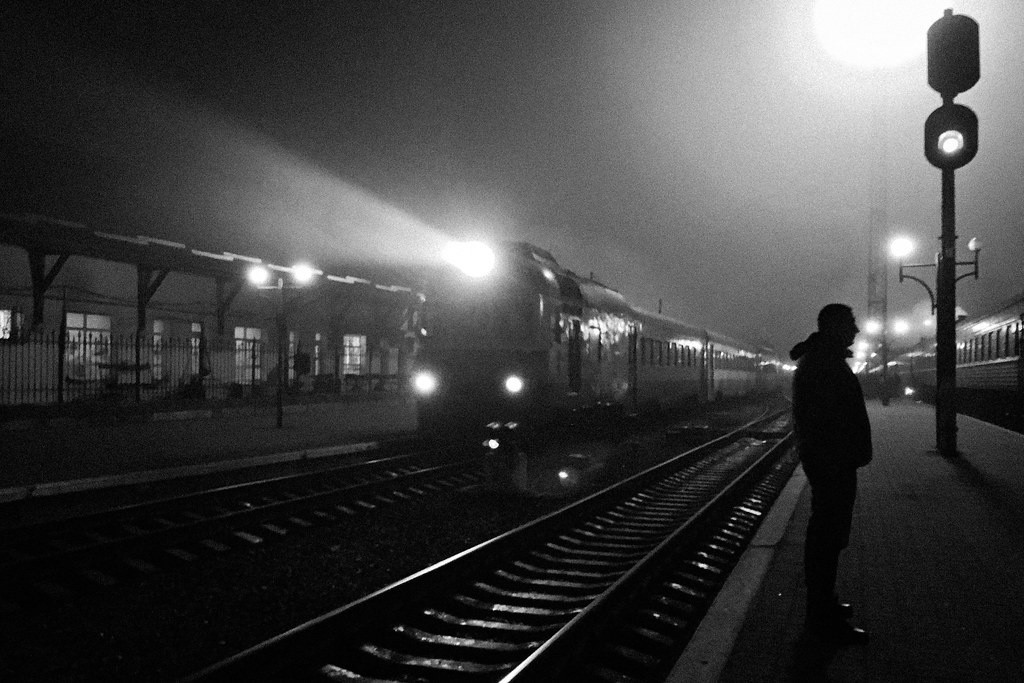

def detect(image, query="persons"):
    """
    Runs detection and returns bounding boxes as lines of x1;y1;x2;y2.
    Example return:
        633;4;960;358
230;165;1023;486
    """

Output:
790;305;876;642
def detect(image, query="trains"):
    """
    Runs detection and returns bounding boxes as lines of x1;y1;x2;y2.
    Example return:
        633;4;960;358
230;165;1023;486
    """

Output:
409;241;794;441
897;290;1024;428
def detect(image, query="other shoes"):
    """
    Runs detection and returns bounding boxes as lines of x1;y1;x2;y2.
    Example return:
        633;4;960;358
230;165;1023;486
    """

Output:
838;603;851;614
805;624;869;644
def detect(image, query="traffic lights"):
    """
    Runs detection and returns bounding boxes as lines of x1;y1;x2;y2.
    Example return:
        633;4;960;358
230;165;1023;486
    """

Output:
925;104;978;170
927;15;981;94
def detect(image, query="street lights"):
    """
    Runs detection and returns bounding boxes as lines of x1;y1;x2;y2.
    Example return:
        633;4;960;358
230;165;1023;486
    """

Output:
869;322;905;406
252;268;310;428
